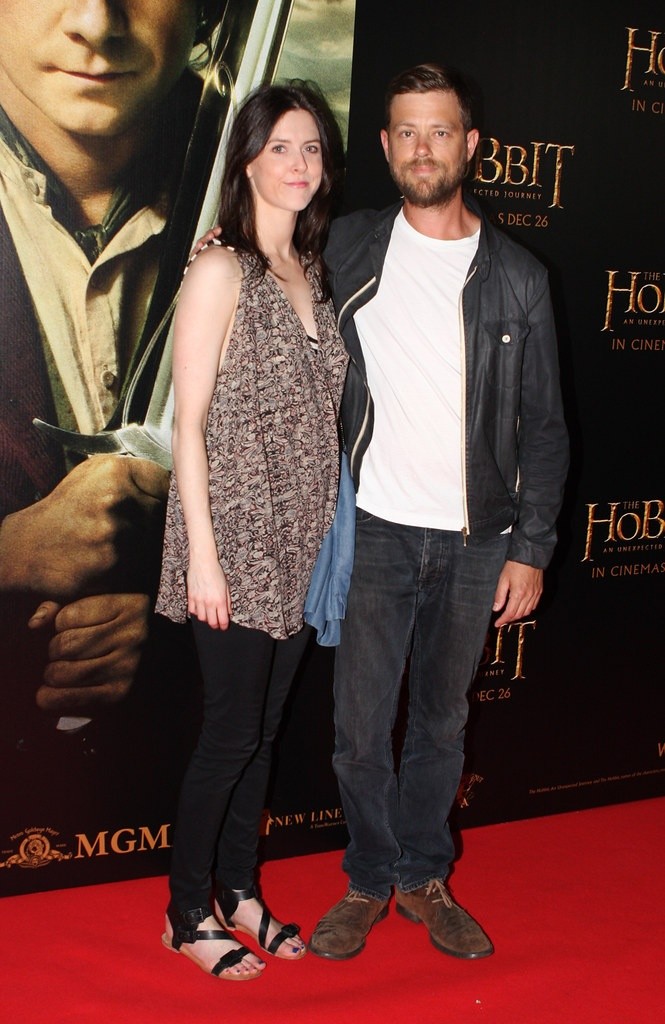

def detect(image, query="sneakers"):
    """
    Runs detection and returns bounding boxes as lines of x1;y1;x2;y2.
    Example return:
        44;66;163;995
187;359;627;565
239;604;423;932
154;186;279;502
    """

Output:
309;886;391;960
394;879;494;958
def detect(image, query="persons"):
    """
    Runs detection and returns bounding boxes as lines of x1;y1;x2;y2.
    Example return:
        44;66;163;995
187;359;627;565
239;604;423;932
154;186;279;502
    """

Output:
152;85;349;979
187;61;565;962
0;0;223;724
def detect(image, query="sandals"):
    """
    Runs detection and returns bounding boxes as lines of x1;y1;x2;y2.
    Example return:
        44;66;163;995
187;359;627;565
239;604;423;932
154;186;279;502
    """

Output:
162;902;263;981
215;886;306;960
